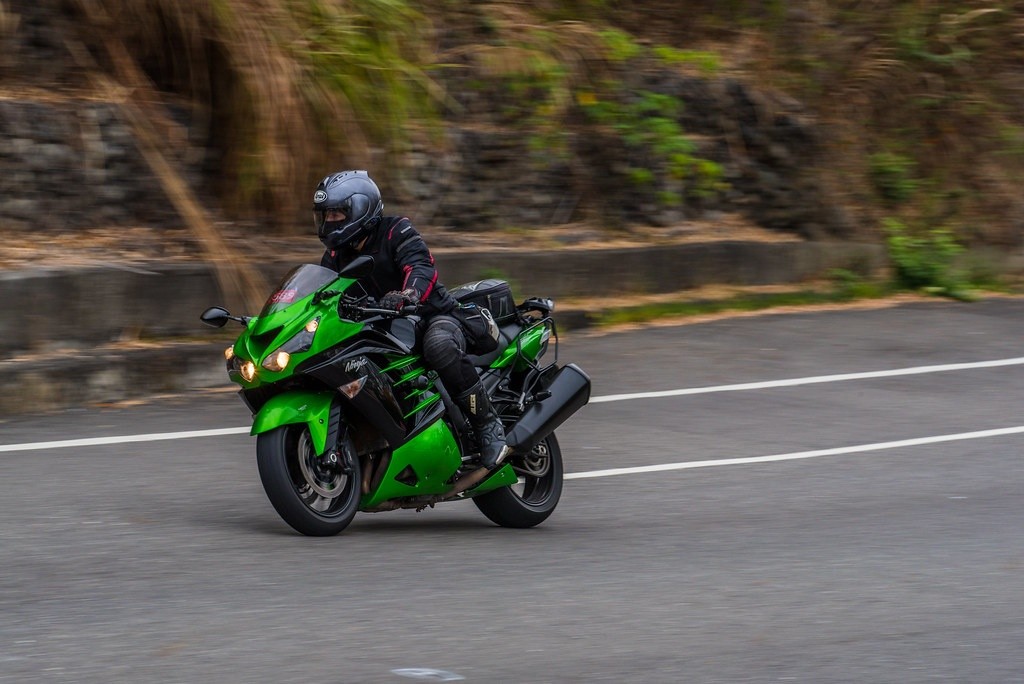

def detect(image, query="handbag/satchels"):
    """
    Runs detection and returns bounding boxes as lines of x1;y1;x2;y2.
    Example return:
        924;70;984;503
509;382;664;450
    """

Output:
458;302;500;356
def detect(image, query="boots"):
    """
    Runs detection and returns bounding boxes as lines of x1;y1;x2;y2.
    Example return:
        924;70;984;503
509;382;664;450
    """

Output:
450;376;509;470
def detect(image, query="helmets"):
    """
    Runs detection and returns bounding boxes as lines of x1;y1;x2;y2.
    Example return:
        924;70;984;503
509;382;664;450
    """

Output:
311;169;385;250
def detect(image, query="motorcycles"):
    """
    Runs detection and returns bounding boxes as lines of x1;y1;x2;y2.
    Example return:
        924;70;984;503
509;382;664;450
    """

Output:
199;255;591;537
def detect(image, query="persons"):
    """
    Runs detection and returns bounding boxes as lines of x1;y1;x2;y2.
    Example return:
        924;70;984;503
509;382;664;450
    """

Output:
312;170;507;470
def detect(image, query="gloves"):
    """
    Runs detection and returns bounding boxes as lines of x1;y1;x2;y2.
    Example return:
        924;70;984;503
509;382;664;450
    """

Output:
378;289;417;320
